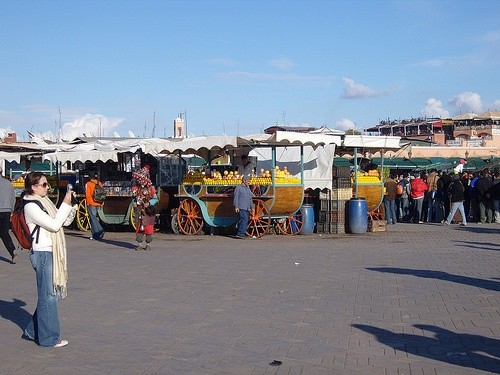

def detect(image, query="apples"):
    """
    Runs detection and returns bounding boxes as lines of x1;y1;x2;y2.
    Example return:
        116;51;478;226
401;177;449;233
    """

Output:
223;170;243;180
17;176;25;182
365;169;379;177
260;165;292;177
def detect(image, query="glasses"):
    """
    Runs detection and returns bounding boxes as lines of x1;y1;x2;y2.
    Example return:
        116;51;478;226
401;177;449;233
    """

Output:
34;182;49;188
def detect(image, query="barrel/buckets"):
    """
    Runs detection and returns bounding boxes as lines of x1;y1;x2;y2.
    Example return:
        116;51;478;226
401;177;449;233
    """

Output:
291;203;315;234
348;197;368;233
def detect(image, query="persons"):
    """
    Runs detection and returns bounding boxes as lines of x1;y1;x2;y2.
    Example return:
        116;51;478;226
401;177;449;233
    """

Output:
0;166;18;264
454;159;467;174
384;168;500;226
234;175;254;238
238;155;254;181
22;172;77;348
131;166;158;251
85;171;105;240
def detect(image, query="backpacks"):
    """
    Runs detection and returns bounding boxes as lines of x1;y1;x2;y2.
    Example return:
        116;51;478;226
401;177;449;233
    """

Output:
10;198;44;249
90;180;106;203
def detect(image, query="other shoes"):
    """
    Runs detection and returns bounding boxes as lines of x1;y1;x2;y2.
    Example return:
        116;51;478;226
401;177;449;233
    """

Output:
145;245;150;250
445;222;449;226
461;223;465;226
12;250;18;264
135;246;142;251
236;235;245;239
53;340;68;347
98;232;105;239
418;220;423;223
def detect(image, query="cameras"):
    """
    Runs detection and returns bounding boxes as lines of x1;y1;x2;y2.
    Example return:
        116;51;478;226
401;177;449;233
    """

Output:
67;184;74;192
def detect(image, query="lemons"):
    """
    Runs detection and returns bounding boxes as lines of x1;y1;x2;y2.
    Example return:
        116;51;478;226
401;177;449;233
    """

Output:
183;177;300;184
357;176;379;182
12;182;25;187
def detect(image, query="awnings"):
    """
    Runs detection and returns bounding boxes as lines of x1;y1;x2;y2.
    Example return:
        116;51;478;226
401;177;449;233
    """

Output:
334;157;500;171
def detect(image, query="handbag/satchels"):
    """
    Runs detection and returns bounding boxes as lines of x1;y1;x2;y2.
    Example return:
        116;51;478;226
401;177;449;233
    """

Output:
396;184;403;194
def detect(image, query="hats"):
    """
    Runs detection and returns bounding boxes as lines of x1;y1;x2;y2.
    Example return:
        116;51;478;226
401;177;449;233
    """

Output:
242;176;251;182
460;160;465;164
133;165;152;184
